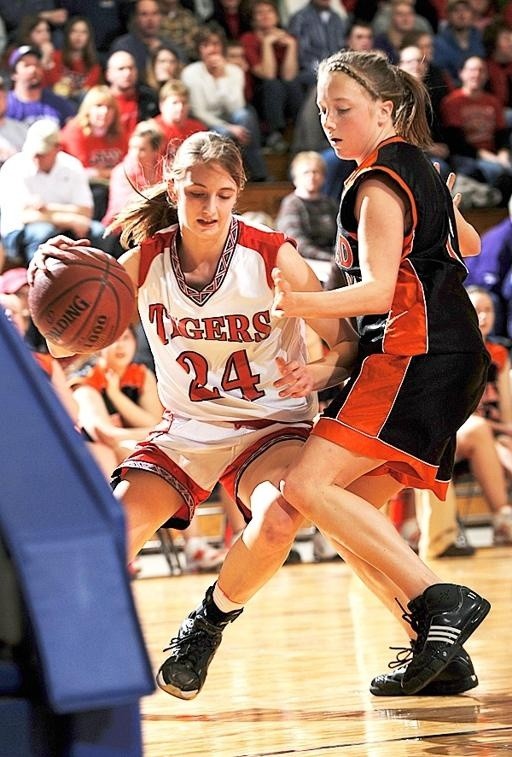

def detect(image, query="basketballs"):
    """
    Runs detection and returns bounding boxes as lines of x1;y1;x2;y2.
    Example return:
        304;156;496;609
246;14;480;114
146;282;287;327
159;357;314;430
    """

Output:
28;248;136;350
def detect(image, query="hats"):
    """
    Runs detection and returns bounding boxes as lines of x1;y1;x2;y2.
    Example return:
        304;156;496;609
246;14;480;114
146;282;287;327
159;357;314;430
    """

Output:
22;119;60;151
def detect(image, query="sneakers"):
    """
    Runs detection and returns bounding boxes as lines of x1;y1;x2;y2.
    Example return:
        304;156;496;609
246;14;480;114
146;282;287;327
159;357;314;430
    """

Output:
158;582;244;701
370;587;491;694
184;543;228;570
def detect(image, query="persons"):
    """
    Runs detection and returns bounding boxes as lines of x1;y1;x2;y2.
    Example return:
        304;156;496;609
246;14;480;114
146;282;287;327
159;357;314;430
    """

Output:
3;266;230;570
283;0;511;263
0;0;296;272
272;52;489;694
25;129;364;700
279;218;512;562
